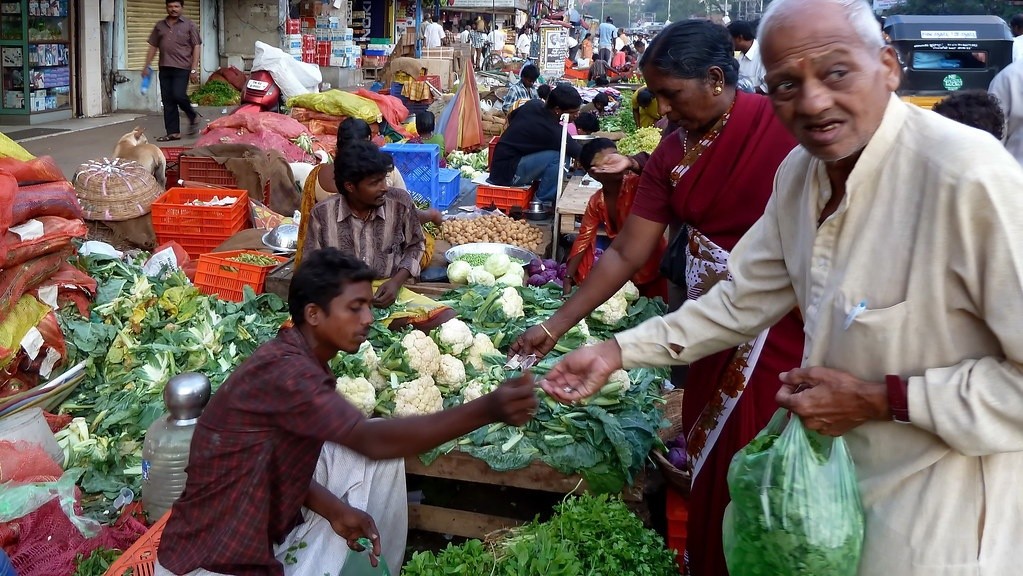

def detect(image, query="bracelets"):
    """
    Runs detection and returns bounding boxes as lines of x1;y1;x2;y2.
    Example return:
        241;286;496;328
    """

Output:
885;375;911;425
626;155;634;170
541;323;557;343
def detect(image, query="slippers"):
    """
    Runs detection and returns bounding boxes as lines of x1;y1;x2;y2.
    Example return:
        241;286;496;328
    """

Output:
188;111;203;134
156;134;181;141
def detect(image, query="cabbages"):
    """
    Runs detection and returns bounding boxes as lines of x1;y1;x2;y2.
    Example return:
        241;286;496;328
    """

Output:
446;253;523;286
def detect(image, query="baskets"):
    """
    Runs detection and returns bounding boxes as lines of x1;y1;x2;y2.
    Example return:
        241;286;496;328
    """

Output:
650;389;693;489
72;157;165;221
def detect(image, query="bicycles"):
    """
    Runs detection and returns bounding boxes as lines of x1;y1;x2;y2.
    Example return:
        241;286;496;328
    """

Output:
471;38;504;72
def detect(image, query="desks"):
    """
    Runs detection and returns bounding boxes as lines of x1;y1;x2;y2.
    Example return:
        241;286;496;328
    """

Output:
404;451;646;546
555;174;600;234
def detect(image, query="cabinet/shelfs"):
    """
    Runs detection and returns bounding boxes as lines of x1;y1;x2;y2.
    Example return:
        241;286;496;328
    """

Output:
0;0;73;124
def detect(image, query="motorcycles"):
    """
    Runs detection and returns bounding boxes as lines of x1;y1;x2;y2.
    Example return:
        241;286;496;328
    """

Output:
879;14;1014;109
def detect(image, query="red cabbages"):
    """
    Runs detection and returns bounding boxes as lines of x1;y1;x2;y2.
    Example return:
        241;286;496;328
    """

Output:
527;247;604;287
664;433;689;472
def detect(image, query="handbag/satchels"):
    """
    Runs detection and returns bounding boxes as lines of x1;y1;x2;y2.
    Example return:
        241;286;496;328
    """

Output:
577;58;589;70
722;406;866;576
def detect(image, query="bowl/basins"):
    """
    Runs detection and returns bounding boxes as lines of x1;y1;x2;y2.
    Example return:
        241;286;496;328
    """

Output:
523;210;549;220
443;242;534;267
261;224;299;252
365;49;385;56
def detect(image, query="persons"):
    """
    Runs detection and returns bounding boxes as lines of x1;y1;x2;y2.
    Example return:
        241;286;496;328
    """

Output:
990;14;1023;166
591;120;679;176
563;138;670;303
541;0;1023;576
420;13;506;68
932;89;1003;141
141;0;202;141
155;110;537;576
502;65;540;113
567;16;649;86
506;20;805;575
489;84;608;202
727;20;769;95
515;28;531;57
631;85;662;129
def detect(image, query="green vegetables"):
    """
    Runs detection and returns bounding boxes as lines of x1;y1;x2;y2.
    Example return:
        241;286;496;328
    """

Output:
70;545;135;576
52;251;292;518
400;490;681;576
189;79;241;105
414;200;435;235
599;75;645;134
726;432;864;576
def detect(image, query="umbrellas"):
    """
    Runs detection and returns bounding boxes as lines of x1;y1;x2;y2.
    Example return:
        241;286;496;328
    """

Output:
437;57;484;153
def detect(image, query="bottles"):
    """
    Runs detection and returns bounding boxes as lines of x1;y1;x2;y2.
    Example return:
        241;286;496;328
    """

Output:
140;66;152;95
142;371;212;525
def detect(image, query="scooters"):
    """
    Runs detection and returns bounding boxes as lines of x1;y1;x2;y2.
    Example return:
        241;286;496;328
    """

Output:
228;70;288;116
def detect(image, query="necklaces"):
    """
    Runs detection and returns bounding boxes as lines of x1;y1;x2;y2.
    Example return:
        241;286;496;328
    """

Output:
420;133;433;139
684;131;688;153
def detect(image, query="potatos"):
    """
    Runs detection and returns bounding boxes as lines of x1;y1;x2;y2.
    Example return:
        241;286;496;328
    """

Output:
434;214;544;250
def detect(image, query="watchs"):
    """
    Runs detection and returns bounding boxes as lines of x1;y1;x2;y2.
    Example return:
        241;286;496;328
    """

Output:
190;69;196;74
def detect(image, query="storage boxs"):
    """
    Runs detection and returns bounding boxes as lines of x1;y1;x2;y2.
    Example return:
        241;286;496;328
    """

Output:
665;484;688;573
151;146;290;305
103;508;172;576
476;135;535;220
1;2;69;111
288;0;442;118
378;142;461;212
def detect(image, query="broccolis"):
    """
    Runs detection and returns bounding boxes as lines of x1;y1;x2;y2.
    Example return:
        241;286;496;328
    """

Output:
445;148;489;178
569;279;639;398
328;286;524;420
